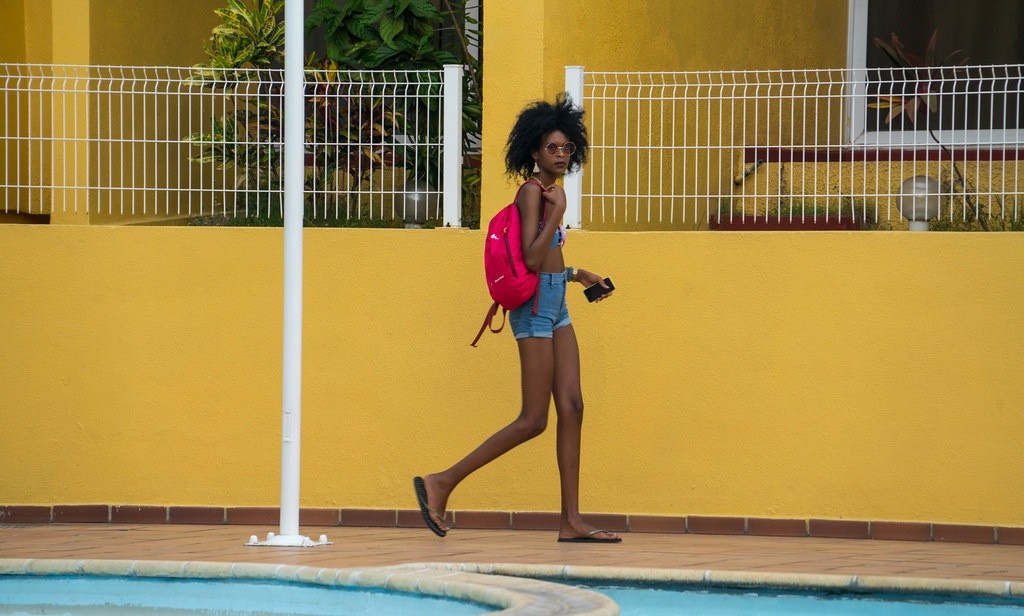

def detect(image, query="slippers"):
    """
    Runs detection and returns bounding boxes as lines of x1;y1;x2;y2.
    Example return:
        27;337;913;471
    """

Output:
414;477;447;537
558;529;622;543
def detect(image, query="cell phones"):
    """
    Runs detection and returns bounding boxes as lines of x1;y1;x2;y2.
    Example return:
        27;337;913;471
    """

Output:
583;276;615;303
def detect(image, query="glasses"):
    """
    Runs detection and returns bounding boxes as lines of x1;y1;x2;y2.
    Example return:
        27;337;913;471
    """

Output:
544;142;576;156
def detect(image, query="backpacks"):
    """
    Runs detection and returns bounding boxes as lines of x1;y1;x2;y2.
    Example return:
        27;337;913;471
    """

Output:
470;178;548;348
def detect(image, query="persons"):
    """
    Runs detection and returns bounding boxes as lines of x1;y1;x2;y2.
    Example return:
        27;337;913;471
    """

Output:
413;91;623;543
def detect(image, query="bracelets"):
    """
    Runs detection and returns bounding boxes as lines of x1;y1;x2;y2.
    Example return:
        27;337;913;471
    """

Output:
571;267;579;282
